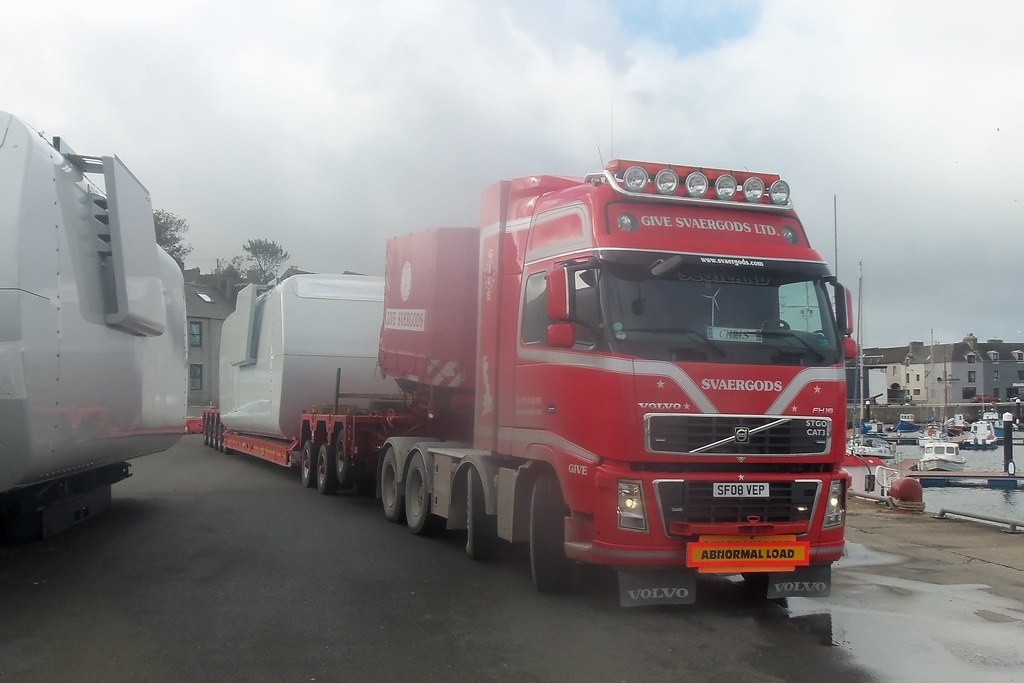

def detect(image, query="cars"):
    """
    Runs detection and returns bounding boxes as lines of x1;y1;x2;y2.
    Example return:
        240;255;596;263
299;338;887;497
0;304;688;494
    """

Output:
1007;393;1024;402
970;395;1000;404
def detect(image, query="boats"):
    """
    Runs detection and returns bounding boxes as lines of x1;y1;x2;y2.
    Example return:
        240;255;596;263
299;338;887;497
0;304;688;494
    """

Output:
847;411;1020;473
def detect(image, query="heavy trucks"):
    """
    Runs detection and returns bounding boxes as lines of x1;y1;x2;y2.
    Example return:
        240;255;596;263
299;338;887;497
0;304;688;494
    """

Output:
199;159;860;614
